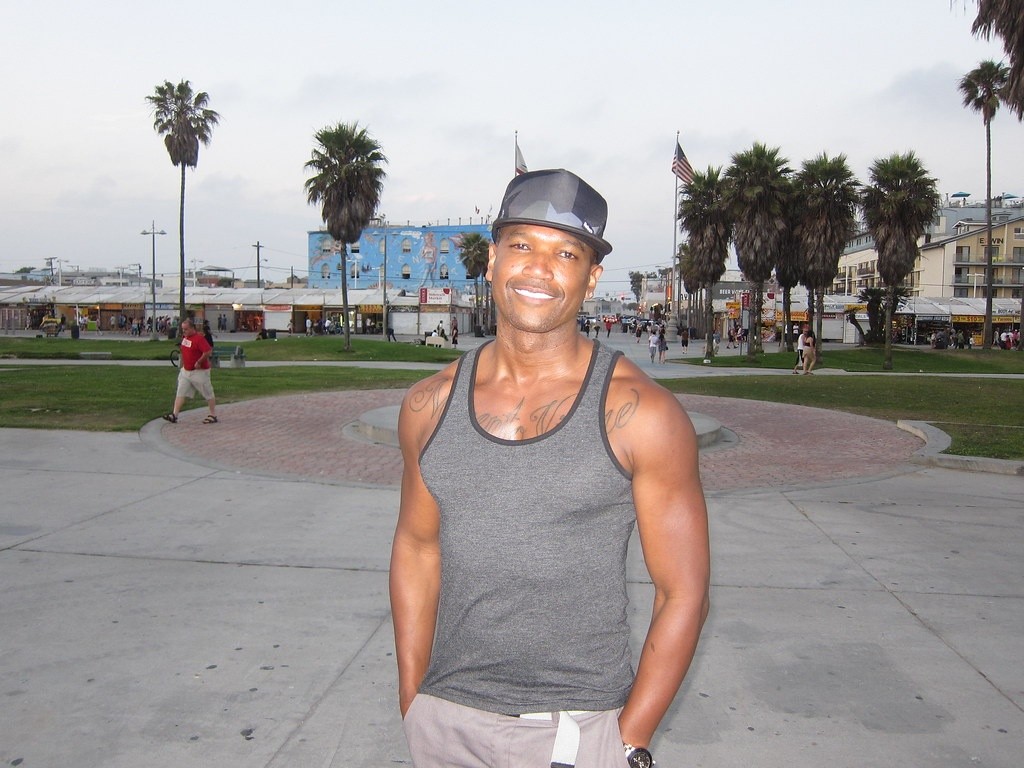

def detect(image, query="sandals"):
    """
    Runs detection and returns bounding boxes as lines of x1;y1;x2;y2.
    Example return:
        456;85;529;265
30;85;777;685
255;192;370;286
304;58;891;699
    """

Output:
162;413;178;423
202;415;218;424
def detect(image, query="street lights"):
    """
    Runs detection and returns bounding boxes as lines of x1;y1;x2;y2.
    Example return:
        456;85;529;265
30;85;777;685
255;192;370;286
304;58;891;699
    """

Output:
967;273;986;299
371;220;400;342
654;265;669;329
139;219;167;342
371;267;381;290
347;259;358;290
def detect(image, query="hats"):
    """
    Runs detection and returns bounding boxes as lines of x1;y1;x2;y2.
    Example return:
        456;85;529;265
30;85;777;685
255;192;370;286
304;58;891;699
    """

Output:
637;323;642;327
493;168;613;256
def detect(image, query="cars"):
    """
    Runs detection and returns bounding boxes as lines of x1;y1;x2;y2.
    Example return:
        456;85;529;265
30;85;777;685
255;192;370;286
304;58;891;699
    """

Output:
584;317;597;325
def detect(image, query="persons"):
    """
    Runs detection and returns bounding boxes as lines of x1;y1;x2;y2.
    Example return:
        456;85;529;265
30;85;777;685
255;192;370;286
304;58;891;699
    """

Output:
109;315;180;334
307;316;332;336
386;328;397;341
992;327;1021;350
630;318;666;364
680;328;689;354
217;314;257;332
23;311;103;335
287;320;293;335
931;329;974;349
432;316;458;348
163;320;218;423
897;327;902;343
785;322;816;375
584;320;611;337
714;326;741;356
365;317;375;334
388;169;710;768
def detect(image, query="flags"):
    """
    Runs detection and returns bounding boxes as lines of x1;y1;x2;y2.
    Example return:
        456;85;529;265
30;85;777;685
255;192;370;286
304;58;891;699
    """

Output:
671;142;694;184
516;145;527;175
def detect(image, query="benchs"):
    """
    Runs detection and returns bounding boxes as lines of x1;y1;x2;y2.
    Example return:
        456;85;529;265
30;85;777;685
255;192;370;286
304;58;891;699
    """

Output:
210;346;246;368
424;337;451;349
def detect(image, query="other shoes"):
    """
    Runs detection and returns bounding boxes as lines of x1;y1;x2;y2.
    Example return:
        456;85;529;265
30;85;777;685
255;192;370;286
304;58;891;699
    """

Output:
792;372;800;374
682;351;687;355
726;346;730;349
803;372;813;375
734;346;737;349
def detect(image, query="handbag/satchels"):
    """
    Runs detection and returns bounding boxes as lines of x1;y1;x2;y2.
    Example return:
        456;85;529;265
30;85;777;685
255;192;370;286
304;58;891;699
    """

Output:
665;346;669;350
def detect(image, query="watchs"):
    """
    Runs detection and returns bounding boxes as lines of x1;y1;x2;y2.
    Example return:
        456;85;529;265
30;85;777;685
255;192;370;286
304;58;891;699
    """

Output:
623;742;656;768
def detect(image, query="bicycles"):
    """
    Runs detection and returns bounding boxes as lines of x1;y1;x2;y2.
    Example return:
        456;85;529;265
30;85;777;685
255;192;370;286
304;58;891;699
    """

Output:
169;342;182;367
